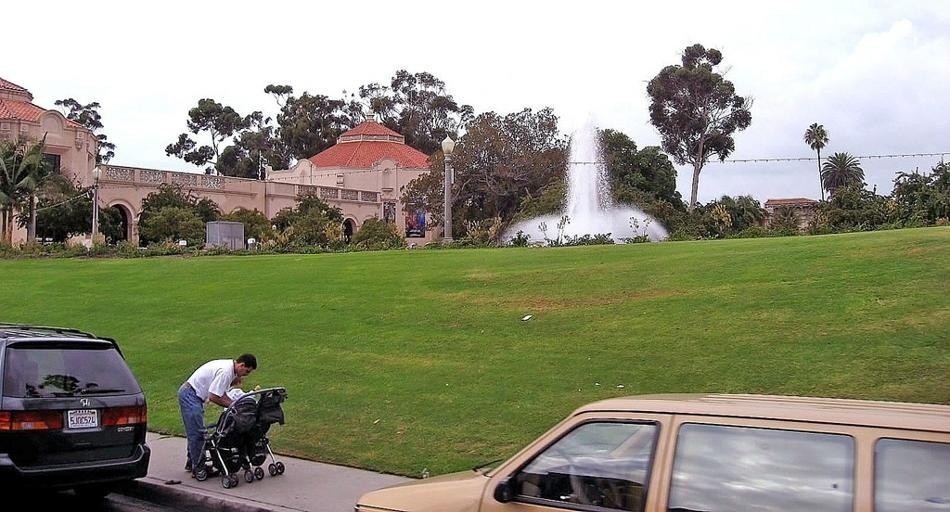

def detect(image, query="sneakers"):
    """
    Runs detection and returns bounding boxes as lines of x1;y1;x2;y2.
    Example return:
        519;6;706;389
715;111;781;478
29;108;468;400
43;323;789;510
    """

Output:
192;469;208;480
184;461;192;471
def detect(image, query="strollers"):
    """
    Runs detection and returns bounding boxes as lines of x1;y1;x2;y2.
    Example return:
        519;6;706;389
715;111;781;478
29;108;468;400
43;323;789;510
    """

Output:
191;386;287;487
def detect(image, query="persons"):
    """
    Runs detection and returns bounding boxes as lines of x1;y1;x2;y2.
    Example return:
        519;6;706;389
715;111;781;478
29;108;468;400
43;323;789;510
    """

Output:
177;353;258;478
223;377;262;412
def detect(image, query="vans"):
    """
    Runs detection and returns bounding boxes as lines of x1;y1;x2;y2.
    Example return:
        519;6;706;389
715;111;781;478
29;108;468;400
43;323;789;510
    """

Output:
354;389;950;511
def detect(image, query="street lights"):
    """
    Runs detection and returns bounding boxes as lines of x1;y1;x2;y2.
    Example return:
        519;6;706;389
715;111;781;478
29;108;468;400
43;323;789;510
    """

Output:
441;133;456;241
90;163;105;232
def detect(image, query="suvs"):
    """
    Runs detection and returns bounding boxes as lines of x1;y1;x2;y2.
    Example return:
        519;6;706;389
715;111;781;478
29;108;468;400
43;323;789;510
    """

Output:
1;322;149;505
